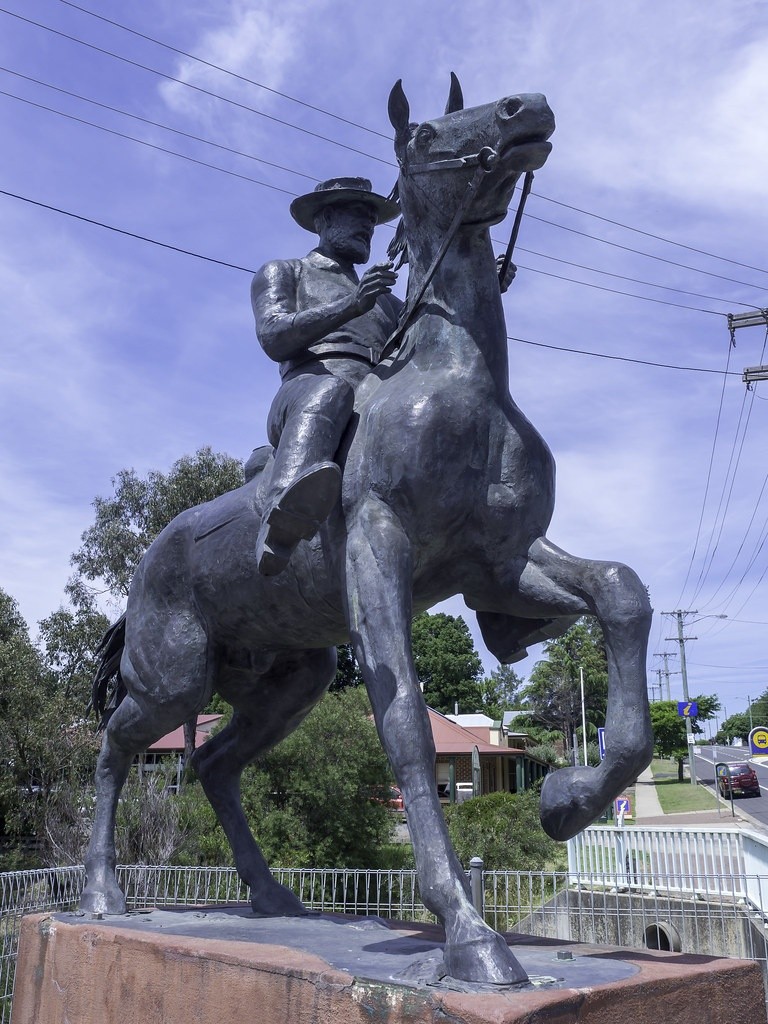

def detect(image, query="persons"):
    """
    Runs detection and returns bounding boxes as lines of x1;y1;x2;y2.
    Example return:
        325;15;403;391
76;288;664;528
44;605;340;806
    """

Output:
250;177;518;575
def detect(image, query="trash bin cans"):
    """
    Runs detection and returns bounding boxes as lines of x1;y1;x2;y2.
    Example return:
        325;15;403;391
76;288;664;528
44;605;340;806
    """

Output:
456;782;473;805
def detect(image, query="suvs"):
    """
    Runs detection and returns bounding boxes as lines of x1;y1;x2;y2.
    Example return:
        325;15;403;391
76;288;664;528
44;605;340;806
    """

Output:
714;761;761;799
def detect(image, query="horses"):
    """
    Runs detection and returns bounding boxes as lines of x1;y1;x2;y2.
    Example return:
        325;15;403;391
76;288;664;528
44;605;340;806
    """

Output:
82;69;661;993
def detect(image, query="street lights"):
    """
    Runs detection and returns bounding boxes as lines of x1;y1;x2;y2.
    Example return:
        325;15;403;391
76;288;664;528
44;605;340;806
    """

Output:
677;613;728;787
